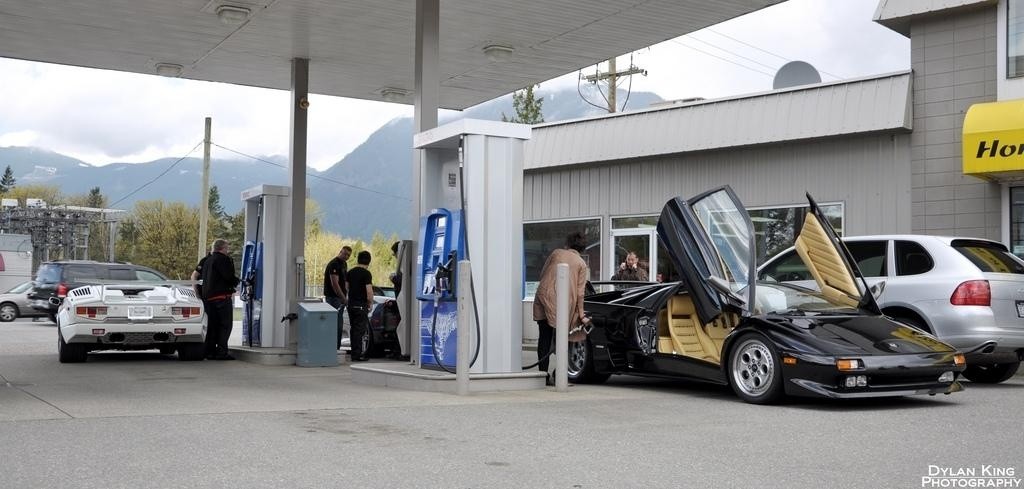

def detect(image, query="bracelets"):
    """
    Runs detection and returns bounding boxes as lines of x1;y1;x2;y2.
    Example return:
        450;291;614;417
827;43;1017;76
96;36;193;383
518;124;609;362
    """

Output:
580;314;587;321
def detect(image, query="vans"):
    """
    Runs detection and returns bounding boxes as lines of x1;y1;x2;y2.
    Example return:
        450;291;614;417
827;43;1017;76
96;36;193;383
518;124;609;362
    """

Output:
29;262;167;326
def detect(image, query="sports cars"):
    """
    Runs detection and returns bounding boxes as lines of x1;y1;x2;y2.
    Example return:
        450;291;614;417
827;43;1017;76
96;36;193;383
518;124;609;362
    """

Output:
55;281;208;364
555;186;968;406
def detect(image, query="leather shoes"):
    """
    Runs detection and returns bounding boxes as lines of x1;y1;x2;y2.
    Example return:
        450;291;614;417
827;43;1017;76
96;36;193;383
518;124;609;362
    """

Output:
220;354;234;360
206;353;215;359
352;354;369;361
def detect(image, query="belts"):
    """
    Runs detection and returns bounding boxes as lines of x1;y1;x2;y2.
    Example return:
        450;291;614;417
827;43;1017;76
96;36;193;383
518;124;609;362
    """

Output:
207;295;226;301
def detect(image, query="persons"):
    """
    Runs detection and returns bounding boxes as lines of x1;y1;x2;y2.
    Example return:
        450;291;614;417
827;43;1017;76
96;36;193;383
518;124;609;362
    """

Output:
201;239;239;361
532;230;590;371
611;248;649;291
344;251;374;362
191;241;216;299
324;245;352;354
656;272;665;284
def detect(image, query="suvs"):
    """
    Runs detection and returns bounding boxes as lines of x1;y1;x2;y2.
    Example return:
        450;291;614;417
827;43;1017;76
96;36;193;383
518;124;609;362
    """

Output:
753;234;1024;385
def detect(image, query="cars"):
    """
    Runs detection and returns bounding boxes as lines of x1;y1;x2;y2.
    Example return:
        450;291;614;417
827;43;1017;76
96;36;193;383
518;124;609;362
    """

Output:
0;281;60;322
330;294;396;341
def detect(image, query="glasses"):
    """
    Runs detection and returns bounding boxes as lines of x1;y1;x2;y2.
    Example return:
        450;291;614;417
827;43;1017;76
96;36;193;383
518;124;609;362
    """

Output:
625;264;633;268
